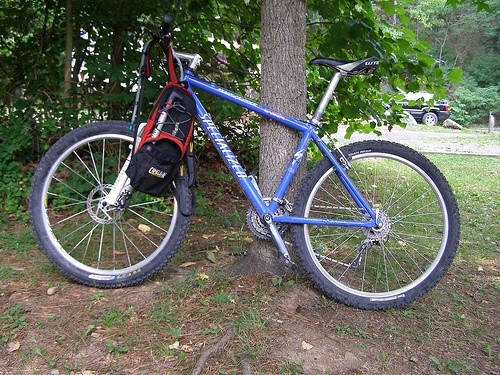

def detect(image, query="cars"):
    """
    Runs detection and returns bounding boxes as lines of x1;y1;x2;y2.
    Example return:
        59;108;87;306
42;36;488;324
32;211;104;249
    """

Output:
374;90;452;127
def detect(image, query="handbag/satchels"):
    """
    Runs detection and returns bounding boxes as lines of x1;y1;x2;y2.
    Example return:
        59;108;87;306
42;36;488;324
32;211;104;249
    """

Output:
125;37;197;197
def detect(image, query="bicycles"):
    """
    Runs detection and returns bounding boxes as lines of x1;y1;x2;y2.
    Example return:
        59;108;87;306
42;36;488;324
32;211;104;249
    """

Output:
28;14;462;311
